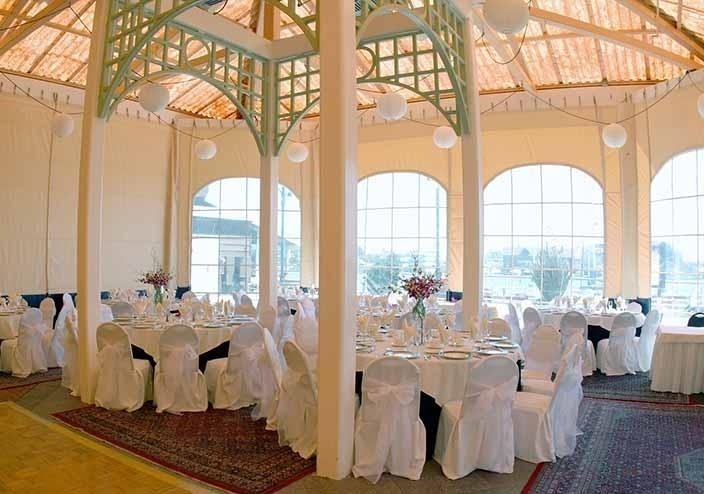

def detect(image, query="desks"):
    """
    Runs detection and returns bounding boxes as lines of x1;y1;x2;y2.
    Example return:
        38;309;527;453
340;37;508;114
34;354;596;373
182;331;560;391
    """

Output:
648;325;703;395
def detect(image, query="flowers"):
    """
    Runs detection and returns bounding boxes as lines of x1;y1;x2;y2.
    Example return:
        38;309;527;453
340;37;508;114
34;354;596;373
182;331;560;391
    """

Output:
134;249;177;289
396;255;453;300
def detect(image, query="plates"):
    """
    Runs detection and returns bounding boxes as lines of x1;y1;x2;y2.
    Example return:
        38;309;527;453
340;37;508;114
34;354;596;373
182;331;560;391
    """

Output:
473;349;507;356
383;350;419;360
485;334;509;341
113;313;158;329
440;350;472;359
493;341;518;349
202;309;253;328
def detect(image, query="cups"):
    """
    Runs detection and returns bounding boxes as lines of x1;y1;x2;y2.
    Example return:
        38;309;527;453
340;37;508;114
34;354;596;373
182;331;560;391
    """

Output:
167;298;192;326
391;329;405;345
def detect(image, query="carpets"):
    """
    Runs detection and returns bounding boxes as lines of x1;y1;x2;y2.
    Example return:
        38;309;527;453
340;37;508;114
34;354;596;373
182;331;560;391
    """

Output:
520;378;703;493
53;395;318;492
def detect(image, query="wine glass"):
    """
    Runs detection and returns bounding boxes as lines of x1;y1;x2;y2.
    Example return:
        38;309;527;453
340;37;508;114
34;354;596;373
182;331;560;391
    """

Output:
414;327;432;363
354;307;412;348
555;296;625;315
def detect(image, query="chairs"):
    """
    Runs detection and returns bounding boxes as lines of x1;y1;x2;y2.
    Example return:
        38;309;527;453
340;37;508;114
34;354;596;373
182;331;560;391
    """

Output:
2;283;662;481
